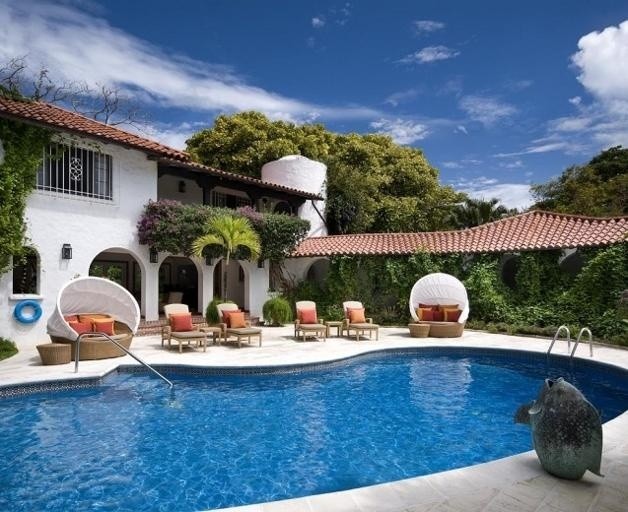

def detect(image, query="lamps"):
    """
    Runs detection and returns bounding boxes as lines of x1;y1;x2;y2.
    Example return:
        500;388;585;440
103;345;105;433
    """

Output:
62;244;72;259
149;246;158;263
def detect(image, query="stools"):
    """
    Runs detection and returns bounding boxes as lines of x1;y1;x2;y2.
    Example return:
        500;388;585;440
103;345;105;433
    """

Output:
408;323;431;338
36;343;71;365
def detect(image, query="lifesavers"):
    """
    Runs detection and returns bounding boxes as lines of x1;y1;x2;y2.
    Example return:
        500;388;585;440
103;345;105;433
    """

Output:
15;301;42;324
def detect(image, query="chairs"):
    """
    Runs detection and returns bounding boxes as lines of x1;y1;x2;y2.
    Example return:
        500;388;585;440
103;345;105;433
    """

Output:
342;301;379;341
160;303;207;353
295;300;327;341
216;303;262;348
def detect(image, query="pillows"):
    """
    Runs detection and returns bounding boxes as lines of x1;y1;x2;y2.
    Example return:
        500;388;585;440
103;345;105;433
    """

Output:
222;309;246;328
64;314;116;337
346;307;365;324
416;303;462;322
168;313;193;332
297;308;317;324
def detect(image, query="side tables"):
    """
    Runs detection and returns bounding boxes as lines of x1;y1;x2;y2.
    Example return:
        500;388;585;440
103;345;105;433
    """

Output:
325;321;342;337
200;327;222;347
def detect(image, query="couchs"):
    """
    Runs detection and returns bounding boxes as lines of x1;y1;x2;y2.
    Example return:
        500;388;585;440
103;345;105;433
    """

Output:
408;272;470;338
50;276;140;360
